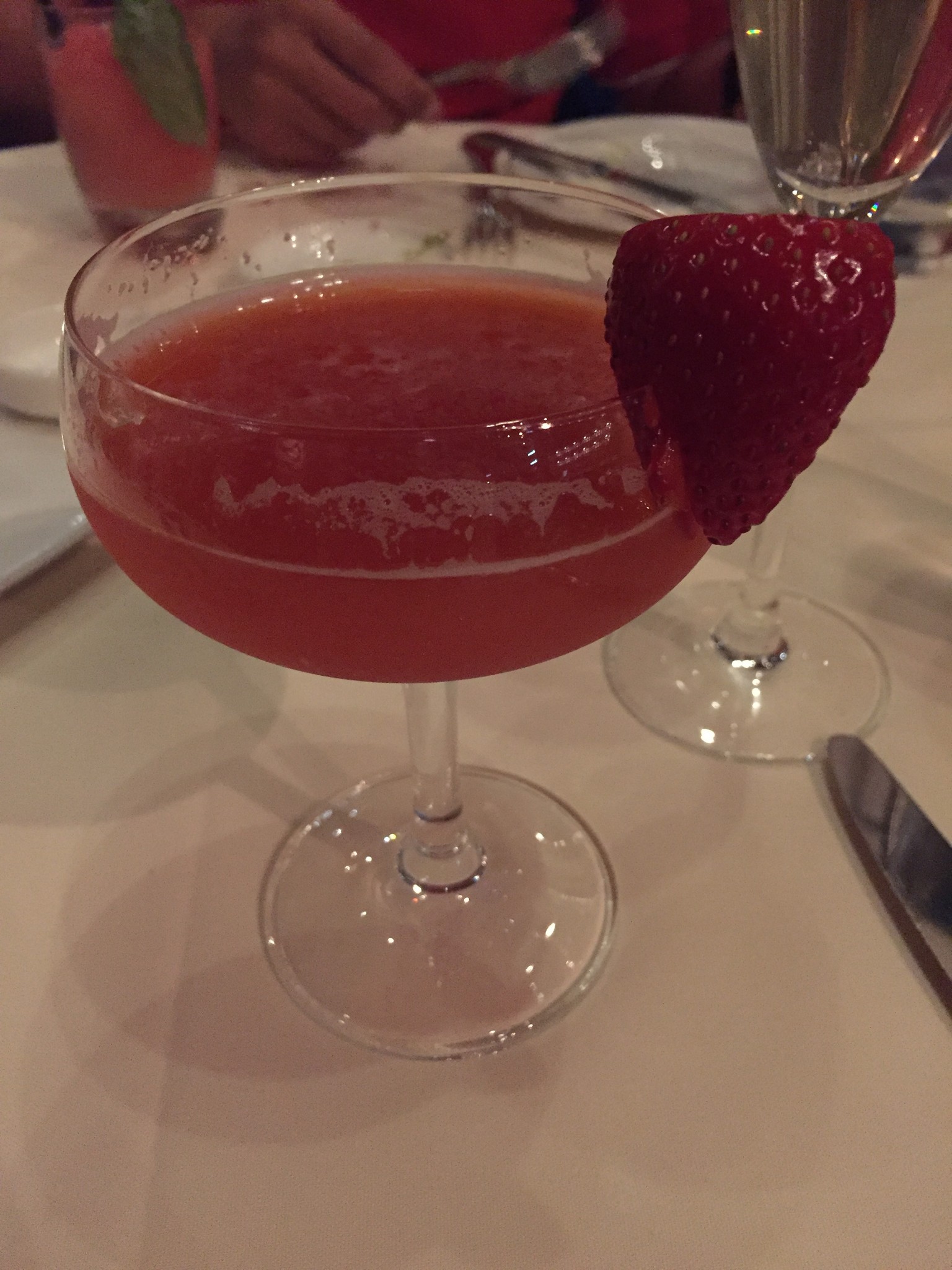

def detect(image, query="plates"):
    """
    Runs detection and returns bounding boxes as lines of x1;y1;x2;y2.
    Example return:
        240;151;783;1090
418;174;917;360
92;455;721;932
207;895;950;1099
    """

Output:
502;111;781;213
0;408;94;596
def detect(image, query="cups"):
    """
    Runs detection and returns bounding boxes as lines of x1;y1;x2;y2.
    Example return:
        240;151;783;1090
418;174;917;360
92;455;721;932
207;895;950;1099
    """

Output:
30;5;218;262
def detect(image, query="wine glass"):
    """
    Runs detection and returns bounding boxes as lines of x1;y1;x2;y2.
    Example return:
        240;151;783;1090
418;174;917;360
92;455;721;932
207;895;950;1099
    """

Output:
608;3;950;766
55;175;709;1061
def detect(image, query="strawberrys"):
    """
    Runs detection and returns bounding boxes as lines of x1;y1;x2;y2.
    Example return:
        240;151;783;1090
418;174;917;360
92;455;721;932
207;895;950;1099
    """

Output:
599;204;898;554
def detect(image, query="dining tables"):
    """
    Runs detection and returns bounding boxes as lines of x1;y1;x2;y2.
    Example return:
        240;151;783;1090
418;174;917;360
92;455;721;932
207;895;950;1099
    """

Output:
1;112;952;1265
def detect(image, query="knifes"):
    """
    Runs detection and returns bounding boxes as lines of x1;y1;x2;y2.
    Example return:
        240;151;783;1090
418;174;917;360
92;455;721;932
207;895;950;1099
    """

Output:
462;128;737;218
824;731;952;984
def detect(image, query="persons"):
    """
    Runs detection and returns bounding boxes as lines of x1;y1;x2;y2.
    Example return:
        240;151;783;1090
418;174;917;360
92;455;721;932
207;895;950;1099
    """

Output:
1;0;734;171
